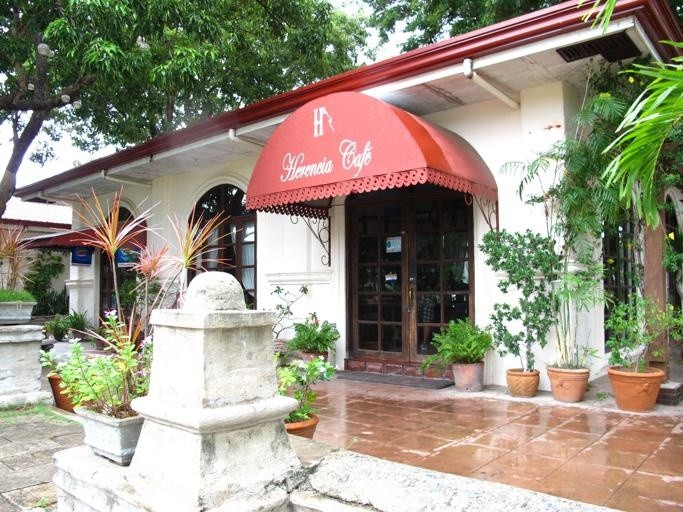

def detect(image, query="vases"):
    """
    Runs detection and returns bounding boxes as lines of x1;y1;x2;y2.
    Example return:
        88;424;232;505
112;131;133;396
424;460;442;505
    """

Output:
73;405;145;468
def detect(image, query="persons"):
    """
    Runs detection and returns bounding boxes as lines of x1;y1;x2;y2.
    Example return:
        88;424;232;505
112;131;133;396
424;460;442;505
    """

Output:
418;272;440;350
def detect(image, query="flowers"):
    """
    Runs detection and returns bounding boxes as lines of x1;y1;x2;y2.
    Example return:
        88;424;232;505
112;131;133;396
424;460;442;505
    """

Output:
58;310;151;418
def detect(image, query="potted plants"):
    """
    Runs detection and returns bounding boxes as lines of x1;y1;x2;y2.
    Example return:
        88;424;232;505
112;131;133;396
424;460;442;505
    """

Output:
605;296;679;410
44;308;115;349
0;288;38;326
521;142;592;404
273;318;339;440
421;316;496;392
479;229;566;398
38;349;93;412
